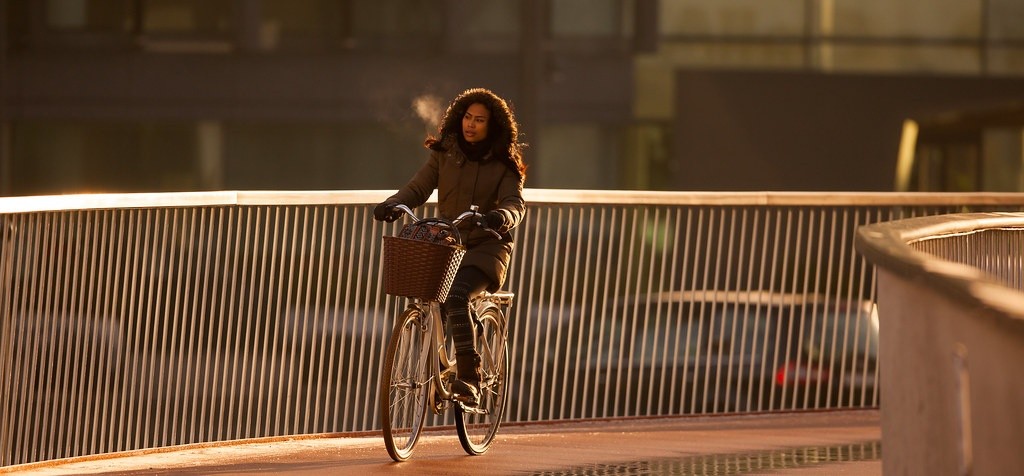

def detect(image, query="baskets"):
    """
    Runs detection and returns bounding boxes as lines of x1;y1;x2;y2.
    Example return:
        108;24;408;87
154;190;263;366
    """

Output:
382;235;466;303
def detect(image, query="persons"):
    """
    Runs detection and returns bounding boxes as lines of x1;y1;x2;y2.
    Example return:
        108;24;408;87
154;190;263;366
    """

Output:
374;87;529;407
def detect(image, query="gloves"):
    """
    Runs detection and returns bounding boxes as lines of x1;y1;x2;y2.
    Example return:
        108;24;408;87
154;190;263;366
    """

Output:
477;210;505;232
373;202;405;223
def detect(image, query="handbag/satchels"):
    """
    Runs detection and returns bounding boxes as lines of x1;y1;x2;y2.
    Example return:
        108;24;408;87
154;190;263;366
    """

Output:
399;224;455;245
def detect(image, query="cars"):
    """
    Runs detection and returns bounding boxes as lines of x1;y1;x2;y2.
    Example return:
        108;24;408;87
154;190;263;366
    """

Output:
512;288;881;423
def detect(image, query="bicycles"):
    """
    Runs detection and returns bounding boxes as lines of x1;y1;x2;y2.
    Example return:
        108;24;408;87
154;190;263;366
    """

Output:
367;202;516;464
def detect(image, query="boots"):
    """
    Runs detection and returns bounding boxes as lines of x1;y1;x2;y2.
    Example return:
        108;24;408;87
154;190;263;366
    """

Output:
451;354;481;405
428;346;448;406
